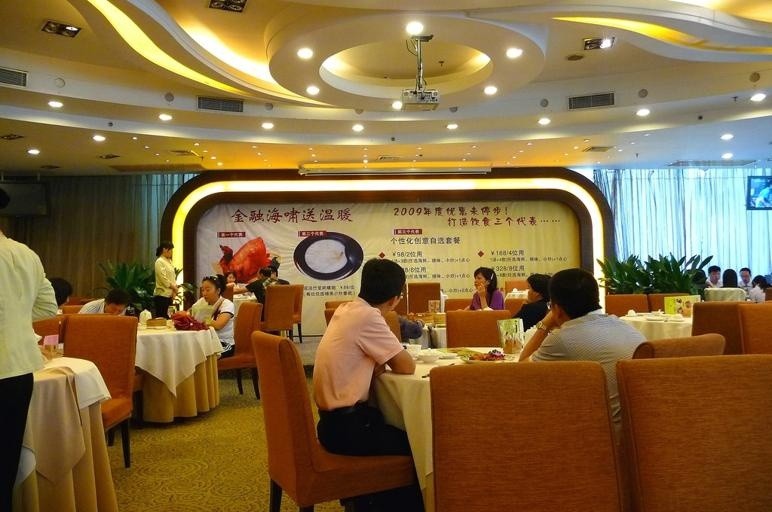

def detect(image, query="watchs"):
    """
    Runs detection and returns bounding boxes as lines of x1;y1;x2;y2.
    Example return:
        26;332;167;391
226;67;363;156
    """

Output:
535;321;548;332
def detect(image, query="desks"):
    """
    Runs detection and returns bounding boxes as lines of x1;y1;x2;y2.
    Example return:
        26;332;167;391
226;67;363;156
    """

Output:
377;346;519;385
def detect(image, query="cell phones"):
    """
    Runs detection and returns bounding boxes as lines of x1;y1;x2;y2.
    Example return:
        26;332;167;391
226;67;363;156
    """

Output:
480;280;491;288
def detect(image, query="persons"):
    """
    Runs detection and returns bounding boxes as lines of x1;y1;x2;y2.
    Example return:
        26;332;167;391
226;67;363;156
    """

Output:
519;269;646;423
398;315;424;344
470;267;503;310
45;241;289;360
0;188;58;512
513;273;550;331
313;259;424;512
755;182;772;207
703;267;766;302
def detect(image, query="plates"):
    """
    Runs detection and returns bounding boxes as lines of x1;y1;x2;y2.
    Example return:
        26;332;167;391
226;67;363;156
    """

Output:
462;356;515;363
443;352;457;358
640;312;685;323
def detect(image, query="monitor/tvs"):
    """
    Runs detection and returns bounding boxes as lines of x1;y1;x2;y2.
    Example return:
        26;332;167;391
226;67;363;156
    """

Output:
746;175;772;210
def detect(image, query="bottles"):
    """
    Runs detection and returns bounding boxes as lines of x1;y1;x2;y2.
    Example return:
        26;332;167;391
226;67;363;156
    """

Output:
675;298;692;317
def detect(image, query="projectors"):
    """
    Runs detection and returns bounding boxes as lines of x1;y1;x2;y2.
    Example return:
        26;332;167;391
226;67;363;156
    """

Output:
400;88;440;113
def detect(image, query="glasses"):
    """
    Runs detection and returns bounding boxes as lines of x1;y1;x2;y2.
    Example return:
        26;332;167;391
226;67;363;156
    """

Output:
546;302;552;309
397;292;404;299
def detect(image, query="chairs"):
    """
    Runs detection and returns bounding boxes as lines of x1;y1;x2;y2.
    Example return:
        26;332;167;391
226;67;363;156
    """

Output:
58;315;138;467
323;281;772;359
425;359;625;510
58;283;304;425
250;327;418;511
615;353;772;511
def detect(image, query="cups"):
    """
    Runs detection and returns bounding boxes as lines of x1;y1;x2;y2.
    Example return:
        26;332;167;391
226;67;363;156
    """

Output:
428;300;441;313
418;351;439;362
